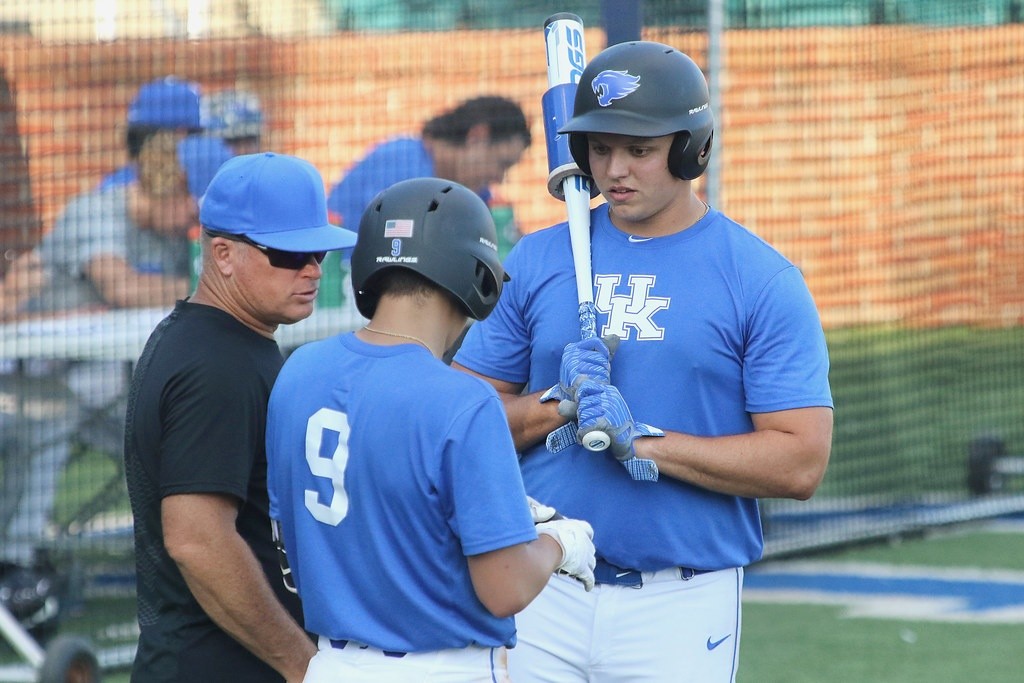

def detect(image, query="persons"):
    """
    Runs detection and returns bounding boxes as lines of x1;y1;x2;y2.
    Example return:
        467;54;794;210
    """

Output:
267;176;597;683
0;78;262;628
453;41;834;683
124;152;358;683
328;96;532;270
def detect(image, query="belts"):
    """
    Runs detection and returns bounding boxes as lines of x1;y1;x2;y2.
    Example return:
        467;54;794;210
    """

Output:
330;639;406;658
594;558;713;589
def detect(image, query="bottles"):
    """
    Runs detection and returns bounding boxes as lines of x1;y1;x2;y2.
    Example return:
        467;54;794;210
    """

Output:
188;227;203;294
316;210;348;307
489;199;514;259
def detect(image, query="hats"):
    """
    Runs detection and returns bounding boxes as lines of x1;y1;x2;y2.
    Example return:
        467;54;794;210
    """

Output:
199;152;358;251
127;80;211;126
202;92;262;139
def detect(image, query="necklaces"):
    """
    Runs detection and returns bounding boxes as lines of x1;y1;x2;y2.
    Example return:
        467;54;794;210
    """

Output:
363;326;431;350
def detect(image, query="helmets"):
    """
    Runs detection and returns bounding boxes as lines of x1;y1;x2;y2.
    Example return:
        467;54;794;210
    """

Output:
558;40;714;180
351;177;511;321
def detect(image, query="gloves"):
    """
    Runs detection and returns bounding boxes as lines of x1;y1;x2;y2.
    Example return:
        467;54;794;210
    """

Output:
526;496;567;526
534;518;597;593
539;334;620;453
557;380;666;482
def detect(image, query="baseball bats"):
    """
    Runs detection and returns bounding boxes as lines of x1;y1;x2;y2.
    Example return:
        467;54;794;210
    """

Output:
543;12;612;453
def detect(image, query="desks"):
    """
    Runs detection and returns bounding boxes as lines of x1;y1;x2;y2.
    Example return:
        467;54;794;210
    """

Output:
0;292;370;564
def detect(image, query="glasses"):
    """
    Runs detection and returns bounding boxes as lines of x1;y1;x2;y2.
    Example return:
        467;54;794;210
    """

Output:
235;235;327;270
